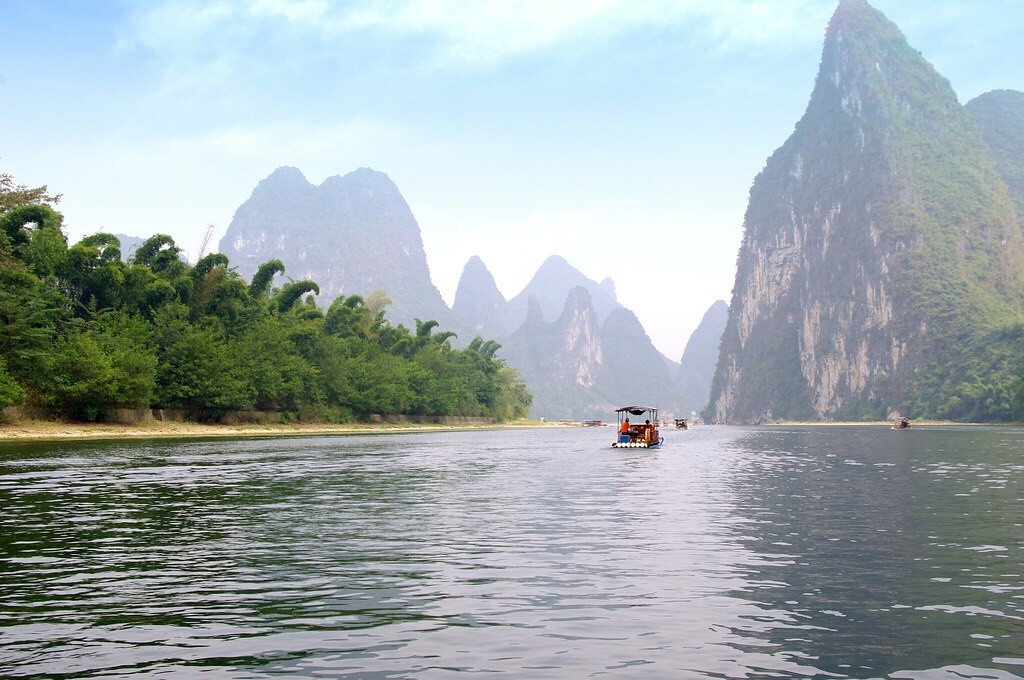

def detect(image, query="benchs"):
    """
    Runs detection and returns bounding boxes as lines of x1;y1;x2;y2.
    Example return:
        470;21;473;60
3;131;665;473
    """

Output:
619;435;629;442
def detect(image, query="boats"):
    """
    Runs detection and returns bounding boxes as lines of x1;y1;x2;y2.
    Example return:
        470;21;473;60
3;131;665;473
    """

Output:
611;405;665;449
896;417;912;429
675;418;689;430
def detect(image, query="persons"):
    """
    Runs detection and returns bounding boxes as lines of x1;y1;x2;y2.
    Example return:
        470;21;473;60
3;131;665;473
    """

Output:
622;418;639;443
